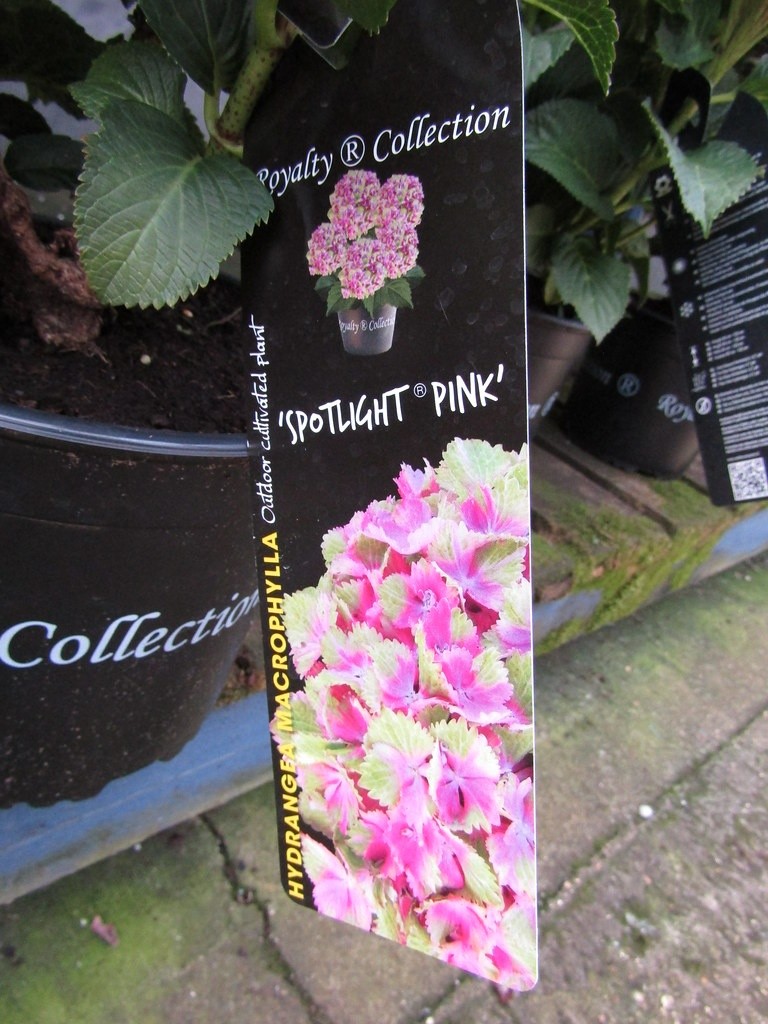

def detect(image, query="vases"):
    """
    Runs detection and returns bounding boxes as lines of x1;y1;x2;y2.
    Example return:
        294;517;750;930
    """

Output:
337;304;396;354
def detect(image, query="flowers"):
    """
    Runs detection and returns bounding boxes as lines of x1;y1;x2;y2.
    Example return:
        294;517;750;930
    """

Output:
272;437;539;992
308;168;425;318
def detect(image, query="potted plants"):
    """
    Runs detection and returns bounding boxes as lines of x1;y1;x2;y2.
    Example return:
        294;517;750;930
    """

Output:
0;0;768;814
